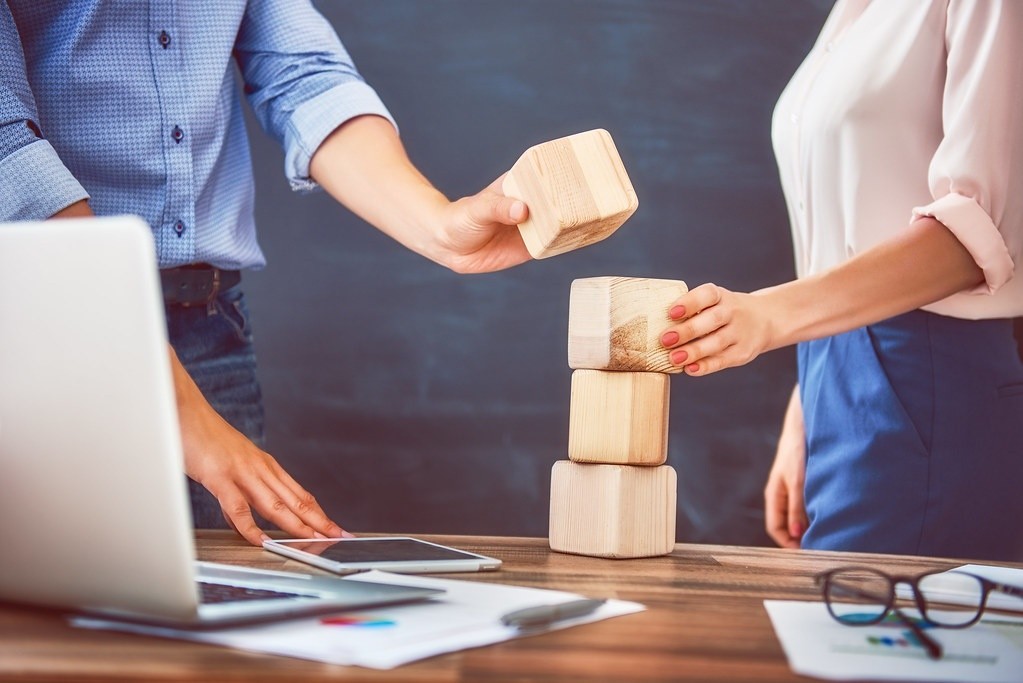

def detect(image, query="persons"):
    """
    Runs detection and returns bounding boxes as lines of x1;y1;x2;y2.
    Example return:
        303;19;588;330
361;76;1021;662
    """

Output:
659;0;1023;563
0;0;536;545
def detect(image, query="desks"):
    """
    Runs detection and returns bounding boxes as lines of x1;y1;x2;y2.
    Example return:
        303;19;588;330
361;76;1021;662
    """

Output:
0;527;1023;683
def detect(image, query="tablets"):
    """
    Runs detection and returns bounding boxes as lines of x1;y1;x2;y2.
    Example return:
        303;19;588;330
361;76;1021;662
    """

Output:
263;536;503;576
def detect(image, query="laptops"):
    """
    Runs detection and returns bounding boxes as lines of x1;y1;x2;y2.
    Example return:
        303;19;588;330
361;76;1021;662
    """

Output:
0;211;449;630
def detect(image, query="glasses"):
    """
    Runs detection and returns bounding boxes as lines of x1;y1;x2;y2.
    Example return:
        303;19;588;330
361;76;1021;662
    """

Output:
814;564;1023;660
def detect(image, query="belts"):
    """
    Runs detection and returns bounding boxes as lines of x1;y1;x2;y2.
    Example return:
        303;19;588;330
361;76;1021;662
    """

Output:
160;270;243;306
